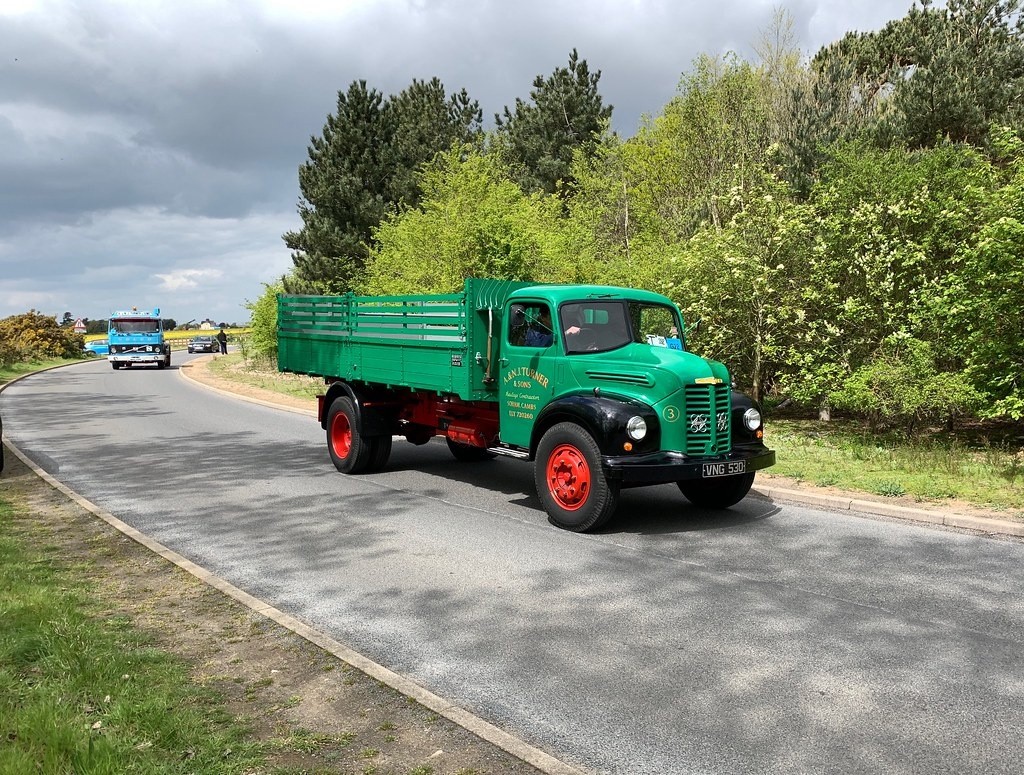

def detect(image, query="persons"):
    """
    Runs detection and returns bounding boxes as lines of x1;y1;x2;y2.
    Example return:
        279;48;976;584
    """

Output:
525;303;580;347
216;329;229;355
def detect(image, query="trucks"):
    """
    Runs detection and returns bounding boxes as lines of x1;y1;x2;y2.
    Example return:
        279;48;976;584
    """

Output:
107;306;171;370
277;277;775;536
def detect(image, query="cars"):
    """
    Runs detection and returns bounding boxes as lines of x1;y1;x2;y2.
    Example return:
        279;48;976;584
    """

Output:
188;336;220;353
82;339;110;356
